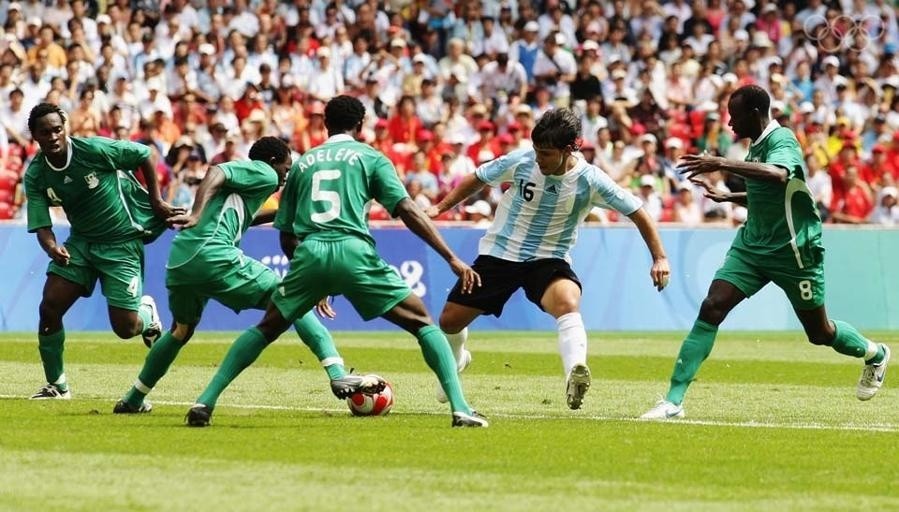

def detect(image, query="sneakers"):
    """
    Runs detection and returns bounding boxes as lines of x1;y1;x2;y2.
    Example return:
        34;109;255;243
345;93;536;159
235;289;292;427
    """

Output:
856;342;891;401
639;398;686;421
29;382;71;401
451;410;489;428
184;405;214;427
564;361;592;411
331;368;388;400
140;295;163;348
113;397;153;414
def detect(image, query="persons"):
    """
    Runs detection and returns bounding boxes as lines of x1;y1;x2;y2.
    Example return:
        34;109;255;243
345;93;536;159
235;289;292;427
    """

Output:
182;95;489;429
114;138;387;416
1;1;899;220
418;113;671;409
25;104;186;397
639;84;890;423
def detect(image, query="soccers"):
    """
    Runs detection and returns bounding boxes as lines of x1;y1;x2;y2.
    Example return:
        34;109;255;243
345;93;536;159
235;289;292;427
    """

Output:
347;375;392;416
